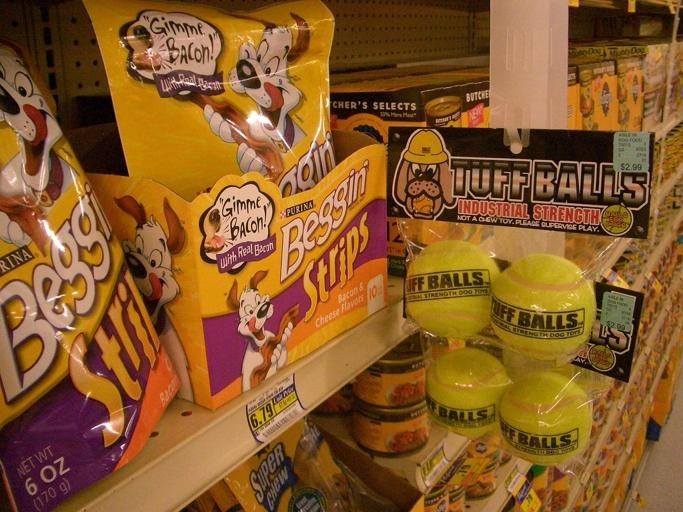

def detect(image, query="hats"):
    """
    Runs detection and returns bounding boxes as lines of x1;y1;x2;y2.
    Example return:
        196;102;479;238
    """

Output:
402;128;449;164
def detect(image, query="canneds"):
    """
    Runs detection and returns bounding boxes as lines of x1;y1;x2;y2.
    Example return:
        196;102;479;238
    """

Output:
425;95;463;127
579;69;593;113
310;332;430;457
